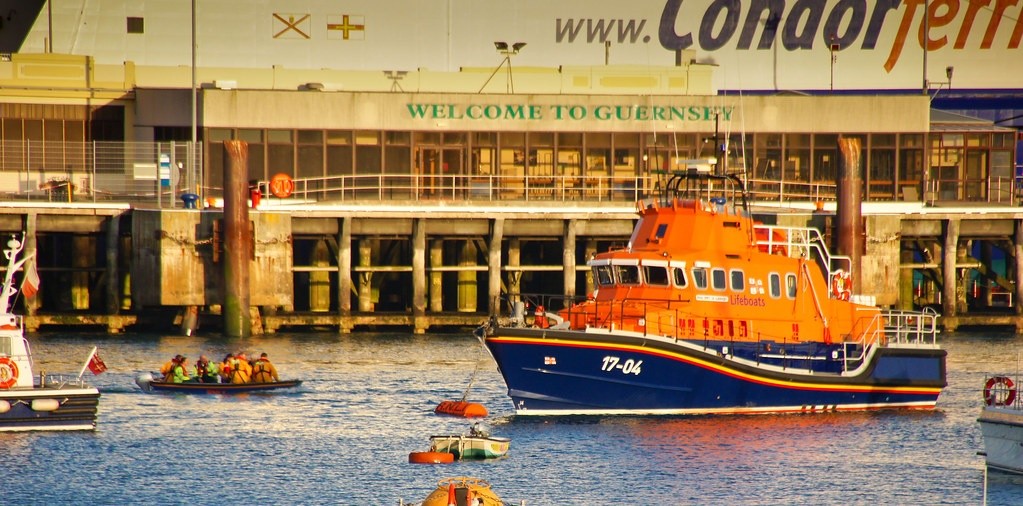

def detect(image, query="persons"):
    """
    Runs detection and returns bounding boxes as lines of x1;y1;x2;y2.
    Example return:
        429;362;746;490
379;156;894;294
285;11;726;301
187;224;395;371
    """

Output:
161;350;278;385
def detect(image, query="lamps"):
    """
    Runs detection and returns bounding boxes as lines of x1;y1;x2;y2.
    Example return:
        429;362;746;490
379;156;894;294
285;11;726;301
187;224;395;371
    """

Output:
925;67;953;96
479;42;529;94
830;44;840;90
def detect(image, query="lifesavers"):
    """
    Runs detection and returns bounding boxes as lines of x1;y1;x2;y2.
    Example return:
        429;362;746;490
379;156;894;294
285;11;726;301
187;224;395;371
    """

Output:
984;377;1016;407
0;358;20;390
833;270;853;301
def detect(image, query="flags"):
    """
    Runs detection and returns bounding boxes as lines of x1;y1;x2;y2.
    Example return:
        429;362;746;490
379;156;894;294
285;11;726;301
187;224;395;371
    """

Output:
22;262;40;298
89;353;107;375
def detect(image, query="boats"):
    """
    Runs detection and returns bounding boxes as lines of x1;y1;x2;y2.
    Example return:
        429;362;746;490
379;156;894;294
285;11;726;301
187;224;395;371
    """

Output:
976;352;1023;475
472;91;948;416
134;370;303;392
0;232;101;432
429;423;512;460
399;477;525;506
